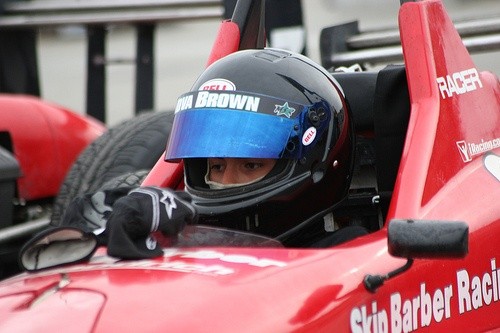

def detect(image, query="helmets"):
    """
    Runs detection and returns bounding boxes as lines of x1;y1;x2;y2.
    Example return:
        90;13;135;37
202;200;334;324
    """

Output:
165;49;356;227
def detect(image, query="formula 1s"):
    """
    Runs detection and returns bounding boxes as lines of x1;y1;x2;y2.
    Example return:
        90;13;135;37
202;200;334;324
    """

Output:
0;2;500;332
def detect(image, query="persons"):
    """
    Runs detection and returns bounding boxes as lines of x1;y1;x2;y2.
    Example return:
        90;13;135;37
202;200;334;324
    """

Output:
165;48;354;250
